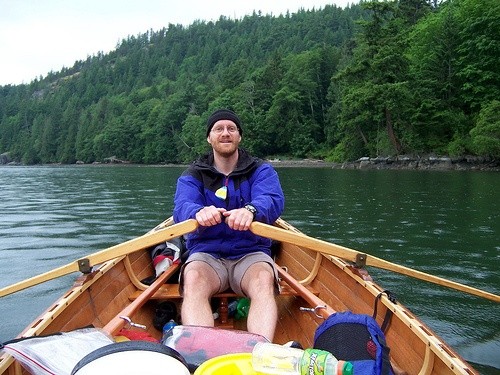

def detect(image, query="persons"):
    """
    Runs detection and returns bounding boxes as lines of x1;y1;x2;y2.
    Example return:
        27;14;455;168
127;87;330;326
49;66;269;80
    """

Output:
173;109;285;345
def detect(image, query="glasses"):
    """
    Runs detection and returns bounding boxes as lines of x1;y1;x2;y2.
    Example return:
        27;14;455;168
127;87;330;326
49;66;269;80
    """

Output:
210;126;239;134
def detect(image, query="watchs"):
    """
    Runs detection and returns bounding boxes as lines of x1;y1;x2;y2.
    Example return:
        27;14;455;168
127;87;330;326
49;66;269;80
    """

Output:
245;205;256;212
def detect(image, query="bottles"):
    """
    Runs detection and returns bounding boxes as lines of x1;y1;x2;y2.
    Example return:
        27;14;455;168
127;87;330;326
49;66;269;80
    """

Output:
252;342;353;375
218;297;228;324
212;300;239;319
162;319;177;342
235;298;251;320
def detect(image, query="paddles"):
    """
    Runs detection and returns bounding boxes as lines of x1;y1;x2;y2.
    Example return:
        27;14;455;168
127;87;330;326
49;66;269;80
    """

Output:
248;221;500;303
0;217;200;299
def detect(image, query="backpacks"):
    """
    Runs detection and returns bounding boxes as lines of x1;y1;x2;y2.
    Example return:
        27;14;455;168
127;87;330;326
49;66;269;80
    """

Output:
312;289;396;375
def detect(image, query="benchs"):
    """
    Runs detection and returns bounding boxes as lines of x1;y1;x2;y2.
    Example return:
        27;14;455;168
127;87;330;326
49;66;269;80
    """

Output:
128;280;320;329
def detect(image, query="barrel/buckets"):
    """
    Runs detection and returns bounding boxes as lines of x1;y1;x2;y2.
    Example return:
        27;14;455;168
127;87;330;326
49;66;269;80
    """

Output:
193;352;273;375
71;341;192;375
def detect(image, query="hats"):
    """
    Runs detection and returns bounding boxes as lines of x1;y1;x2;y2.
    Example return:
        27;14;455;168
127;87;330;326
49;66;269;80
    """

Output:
207;108;243;137
